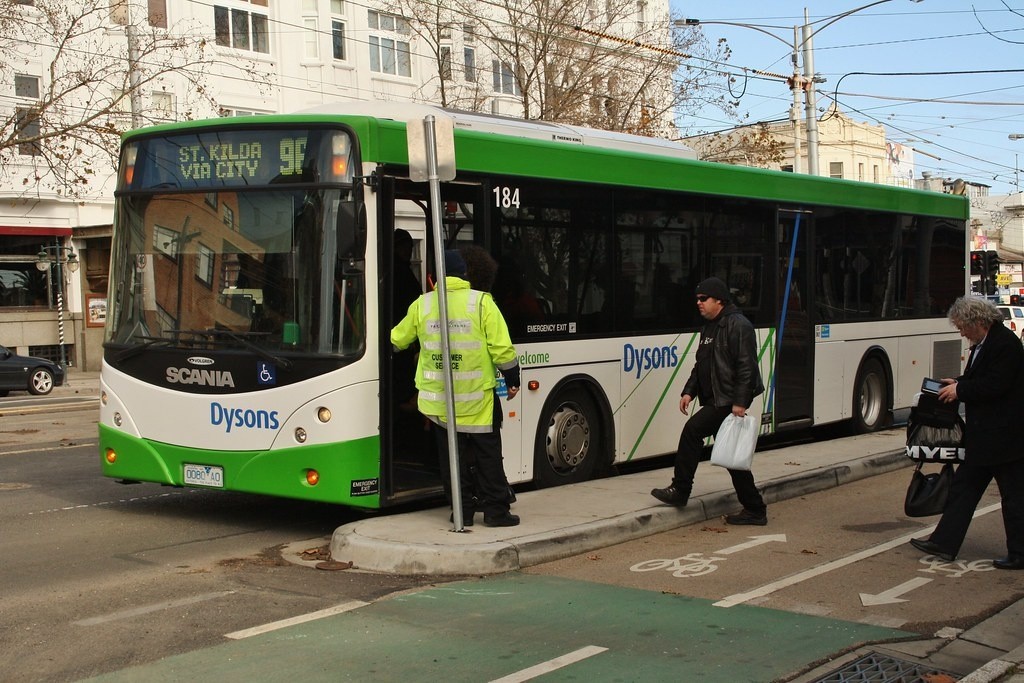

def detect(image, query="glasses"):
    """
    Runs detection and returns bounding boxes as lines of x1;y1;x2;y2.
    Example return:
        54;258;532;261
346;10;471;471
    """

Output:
695;296;710;302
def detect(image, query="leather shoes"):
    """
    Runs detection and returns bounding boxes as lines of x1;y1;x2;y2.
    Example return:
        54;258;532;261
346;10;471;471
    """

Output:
910;538;956;561
993;556;1024;570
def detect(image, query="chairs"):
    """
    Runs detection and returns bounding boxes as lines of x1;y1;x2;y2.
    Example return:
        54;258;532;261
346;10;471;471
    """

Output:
508;295;553;327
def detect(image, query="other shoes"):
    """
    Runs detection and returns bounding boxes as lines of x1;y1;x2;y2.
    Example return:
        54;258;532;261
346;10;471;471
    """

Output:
727;509;768;525
651;482;687;506
484;511;520;525
450;512;473;526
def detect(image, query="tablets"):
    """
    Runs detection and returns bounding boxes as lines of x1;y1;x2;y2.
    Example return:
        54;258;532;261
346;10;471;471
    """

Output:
922;377;948;394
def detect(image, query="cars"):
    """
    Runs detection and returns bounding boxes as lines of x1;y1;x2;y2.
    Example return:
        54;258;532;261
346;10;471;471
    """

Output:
996;305;1024;347
0;345;64;397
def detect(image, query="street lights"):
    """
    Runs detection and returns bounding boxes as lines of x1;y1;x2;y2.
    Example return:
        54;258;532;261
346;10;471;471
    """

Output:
675;0;919;176
34;239;80;382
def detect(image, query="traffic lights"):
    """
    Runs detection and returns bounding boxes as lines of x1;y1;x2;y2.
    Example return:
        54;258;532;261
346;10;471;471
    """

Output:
971;251;999;295
1011;294;1024;306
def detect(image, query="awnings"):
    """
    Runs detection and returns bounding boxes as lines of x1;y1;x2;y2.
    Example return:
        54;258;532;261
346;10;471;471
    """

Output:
0;225;72;236
996;250;1024;262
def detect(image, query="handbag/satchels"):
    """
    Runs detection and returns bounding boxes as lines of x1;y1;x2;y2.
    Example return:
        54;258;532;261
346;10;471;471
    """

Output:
905;464;954;517
905;393;966;464
709;409;759;471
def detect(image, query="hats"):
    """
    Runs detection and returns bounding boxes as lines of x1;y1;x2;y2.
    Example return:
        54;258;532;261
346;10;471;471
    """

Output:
432;251;466;284
696;276;729;300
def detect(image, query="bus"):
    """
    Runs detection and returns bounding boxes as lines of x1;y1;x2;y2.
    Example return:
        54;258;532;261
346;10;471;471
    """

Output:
99;114;973;510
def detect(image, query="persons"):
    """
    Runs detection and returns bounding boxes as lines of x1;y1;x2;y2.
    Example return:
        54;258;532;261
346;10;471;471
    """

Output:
393;228;424;458
650;277;768;526
909;295;1024;571
389;250;520;528
455;242;516;512
